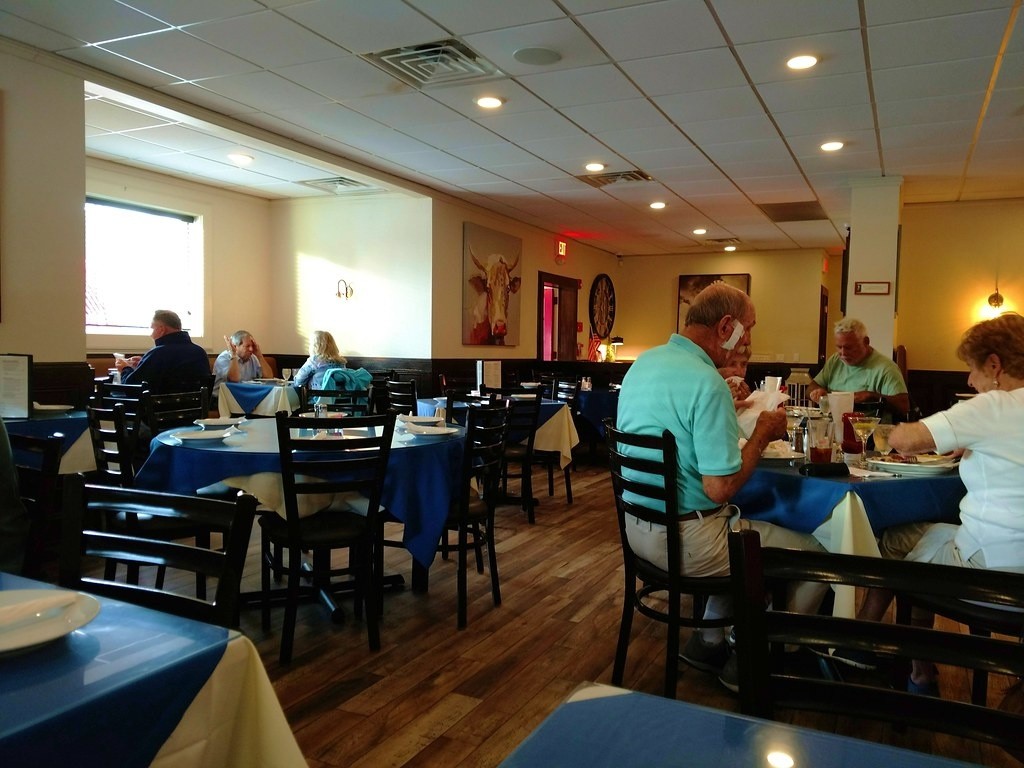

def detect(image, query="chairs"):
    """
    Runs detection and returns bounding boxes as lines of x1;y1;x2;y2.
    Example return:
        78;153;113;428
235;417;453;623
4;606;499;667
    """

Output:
0;357;1023;768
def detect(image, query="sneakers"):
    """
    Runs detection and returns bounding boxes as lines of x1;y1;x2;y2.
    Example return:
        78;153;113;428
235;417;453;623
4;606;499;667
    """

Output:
718;654;747;693
678;631;732;674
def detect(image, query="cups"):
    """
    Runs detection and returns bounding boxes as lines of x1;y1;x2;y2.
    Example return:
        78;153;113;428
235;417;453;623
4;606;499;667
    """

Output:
314;405;327;436
118;362;135;383
108;369;118;382
808;421;835;464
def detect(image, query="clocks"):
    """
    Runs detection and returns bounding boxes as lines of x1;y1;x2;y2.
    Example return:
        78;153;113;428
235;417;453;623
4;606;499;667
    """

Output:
588;274;616;338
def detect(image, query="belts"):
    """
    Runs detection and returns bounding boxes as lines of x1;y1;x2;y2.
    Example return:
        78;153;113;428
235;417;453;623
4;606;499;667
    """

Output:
679;505;723;522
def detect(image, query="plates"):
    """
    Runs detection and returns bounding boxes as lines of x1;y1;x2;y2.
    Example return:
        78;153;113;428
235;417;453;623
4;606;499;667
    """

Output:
242;379;294;386
521;383;541;389
170;430;231;444
193;418;246;429
0;589;101;651
760;453;806;463
299;412;347;418
866;455;960;474
405;428;458;437
399;417;444;426
34;405;74;413
511;394;536;400
433;398;448;403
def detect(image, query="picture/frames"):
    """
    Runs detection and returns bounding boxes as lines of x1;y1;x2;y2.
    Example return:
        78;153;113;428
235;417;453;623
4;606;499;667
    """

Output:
677;274;752;333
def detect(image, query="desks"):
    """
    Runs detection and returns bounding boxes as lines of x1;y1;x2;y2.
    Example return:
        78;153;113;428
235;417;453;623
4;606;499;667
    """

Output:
214;375;376;411
134;417;474;622
708;422;993;704
497;676;974;767
412;395;572;505
2;571;315;767
1;406;120;489
508;379;621;466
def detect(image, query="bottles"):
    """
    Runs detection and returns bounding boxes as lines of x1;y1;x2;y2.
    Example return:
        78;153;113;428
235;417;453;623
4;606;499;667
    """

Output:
793;428;804;463
582;377;591;391
842;413;864;460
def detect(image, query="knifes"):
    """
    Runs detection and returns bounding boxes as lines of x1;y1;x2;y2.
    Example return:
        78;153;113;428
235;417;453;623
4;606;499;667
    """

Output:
919;456;962;465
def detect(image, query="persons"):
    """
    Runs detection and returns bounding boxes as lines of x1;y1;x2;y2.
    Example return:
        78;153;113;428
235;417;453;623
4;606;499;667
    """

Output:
806;313;1024;699
115;309;213;476
805;317;911;425
716;346;751;401
616;280;831;694
211;330;274;418
293;331;345;418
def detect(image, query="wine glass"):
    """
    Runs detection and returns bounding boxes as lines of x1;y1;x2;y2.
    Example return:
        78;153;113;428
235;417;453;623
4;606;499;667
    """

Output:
819;396;832;422
282;369;300;387
786;408;805;450
849;417;896;466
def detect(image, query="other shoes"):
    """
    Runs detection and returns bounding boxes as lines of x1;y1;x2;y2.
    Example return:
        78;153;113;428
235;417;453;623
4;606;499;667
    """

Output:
875;655;940;697
820;647;875;683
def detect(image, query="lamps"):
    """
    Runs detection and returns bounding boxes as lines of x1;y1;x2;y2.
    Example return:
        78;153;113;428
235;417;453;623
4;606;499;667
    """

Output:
611;336;625;360
988;273;1005;309
336;279;353;301
